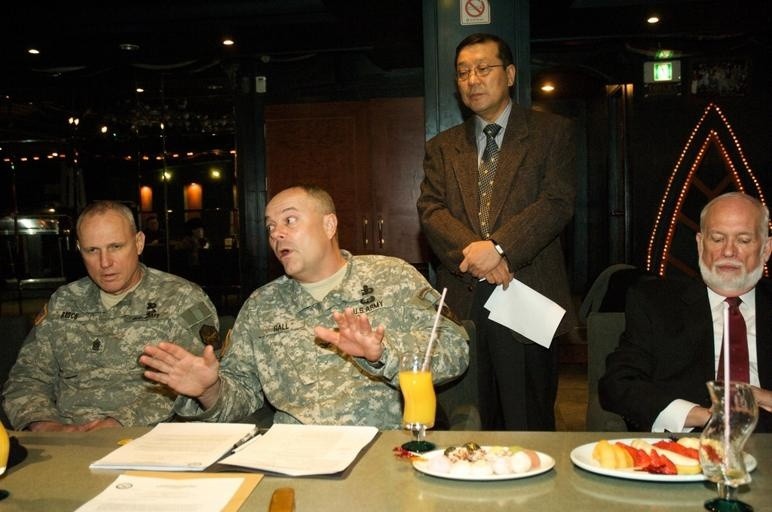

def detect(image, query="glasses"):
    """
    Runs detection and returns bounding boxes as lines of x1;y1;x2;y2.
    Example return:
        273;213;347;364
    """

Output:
457;64;506;79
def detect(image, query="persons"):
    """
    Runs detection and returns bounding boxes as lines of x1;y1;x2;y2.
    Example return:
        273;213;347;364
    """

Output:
135;184;474;434
0;194;221;439
597;188;771;438
417;30;582;429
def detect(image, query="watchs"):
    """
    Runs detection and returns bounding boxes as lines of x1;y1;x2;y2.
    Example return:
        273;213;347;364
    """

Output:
487;236;506;260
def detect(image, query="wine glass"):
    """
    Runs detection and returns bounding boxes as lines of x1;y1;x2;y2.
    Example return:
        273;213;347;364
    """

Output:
694;379;758;512
396;348;438;455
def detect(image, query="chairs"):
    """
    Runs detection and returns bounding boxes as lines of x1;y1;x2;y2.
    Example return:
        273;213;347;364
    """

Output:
436;319;485;431
584;311;637;432
212;312;235;363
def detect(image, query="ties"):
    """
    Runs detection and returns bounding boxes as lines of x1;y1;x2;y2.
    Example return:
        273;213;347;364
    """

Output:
476;122;503;241
717;296;753;386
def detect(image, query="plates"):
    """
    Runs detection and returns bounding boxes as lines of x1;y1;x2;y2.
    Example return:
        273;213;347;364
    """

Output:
413;445;556;480
569;436;755;481
